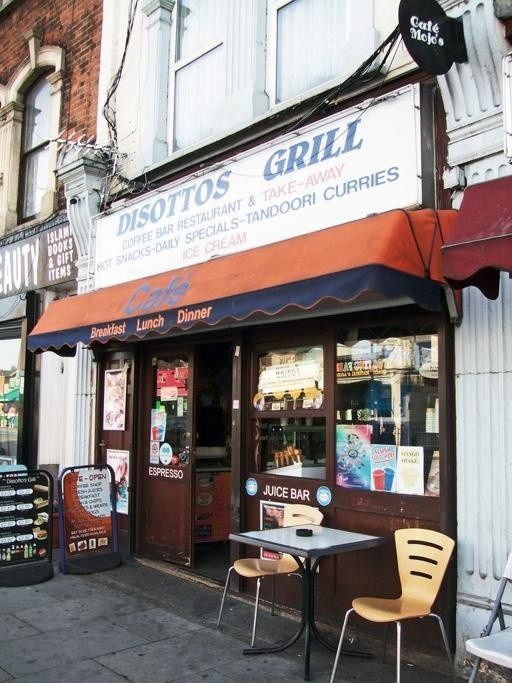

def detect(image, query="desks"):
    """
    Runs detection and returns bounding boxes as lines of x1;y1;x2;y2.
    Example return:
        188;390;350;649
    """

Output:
229;524;388;681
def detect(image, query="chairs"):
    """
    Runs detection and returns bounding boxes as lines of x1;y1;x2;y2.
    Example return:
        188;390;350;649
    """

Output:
464;552;512;682
217;504;324;648
330;528;456;683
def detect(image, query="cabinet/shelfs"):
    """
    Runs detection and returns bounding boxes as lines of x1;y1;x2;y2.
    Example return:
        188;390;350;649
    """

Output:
251;342;324;482
337;338;438;497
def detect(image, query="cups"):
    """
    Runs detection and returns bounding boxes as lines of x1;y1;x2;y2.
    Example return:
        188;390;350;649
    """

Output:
152;427;159;440
384;468;395;491
373;468;384;489
159;425;164;440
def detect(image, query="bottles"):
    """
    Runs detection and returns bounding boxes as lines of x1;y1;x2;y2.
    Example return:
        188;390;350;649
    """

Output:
0;542;37;563
